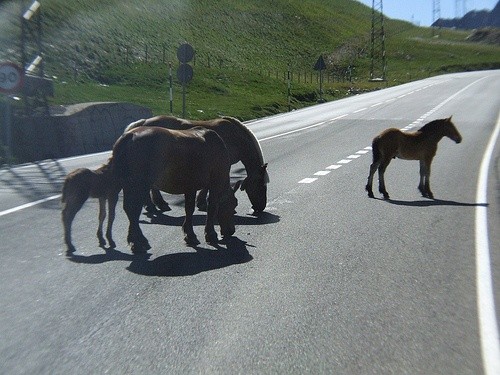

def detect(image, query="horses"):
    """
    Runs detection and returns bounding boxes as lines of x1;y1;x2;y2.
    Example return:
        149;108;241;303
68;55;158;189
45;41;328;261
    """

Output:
59;115;270;256
365;116;463;200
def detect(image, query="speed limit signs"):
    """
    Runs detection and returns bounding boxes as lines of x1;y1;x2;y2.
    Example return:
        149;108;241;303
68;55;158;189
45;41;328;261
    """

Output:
0;63;23;93
